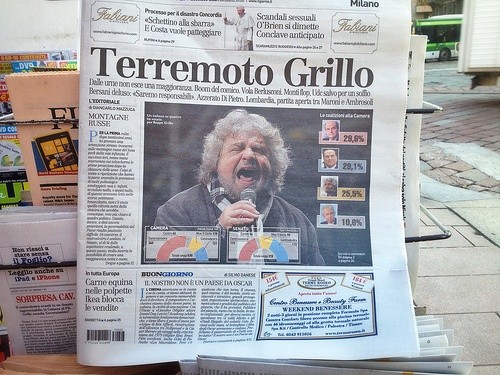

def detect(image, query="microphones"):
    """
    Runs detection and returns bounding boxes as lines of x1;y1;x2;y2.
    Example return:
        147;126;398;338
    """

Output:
241;188;257;232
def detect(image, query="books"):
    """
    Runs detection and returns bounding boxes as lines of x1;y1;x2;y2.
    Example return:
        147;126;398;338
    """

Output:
0;50;80;206
0;306;9;335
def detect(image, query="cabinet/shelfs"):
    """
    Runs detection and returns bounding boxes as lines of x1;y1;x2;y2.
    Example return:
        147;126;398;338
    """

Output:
0;102;451;375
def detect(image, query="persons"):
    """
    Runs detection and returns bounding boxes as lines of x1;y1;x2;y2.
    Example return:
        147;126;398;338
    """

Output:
223;6;255;51
323;149;338;169
320;205;337;225
323;121;339;141
323;177;337;197
153;108;326;266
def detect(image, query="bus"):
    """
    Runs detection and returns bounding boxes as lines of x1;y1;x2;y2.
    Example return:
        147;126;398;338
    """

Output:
411;13;464;62
